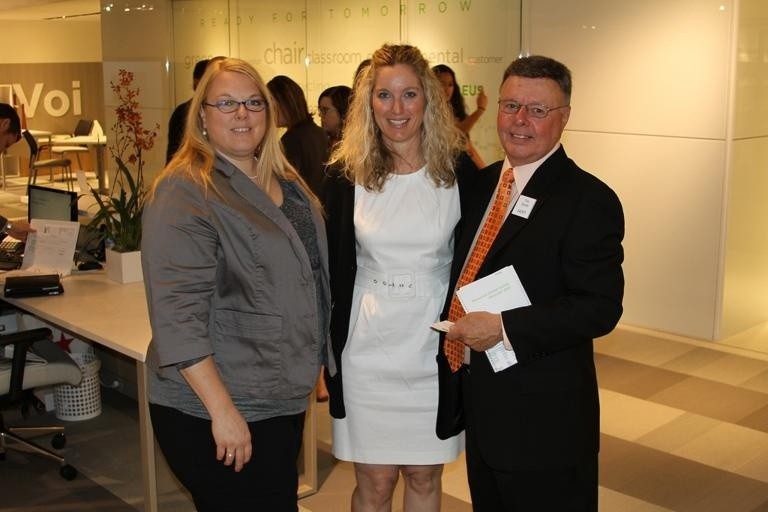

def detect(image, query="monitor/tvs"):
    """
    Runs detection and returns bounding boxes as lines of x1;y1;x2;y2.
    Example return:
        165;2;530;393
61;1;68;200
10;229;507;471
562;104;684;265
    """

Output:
28;185;78;224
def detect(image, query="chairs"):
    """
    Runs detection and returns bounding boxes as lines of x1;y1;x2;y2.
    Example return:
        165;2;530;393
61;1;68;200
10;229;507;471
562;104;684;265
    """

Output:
49;119;93;182
0;149;21;190
22;130;74;195
0;327;82;480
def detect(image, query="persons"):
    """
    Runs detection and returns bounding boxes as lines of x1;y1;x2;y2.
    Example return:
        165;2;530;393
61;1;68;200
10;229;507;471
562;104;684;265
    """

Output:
429;64;488;169
266;74;330;197
434;55;625;512
317;86;352;145
164;60;210;167
319;43;482;512
0;102;37;243
139;56;337;512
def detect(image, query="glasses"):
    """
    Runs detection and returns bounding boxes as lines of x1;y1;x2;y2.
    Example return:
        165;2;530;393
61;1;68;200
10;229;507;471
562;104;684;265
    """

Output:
204;98;269;114
498;99;568;118
318;106;337;115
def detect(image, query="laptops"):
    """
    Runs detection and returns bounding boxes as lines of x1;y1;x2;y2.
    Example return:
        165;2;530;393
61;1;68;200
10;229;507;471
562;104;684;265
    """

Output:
75;119;93;137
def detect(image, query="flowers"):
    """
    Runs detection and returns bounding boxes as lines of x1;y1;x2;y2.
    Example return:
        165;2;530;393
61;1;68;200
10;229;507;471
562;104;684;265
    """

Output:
95;66;161;248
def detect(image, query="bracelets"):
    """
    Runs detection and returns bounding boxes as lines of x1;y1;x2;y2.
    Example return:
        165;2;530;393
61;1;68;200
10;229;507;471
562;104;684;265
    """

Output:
477;106;485;112
2;220;12;235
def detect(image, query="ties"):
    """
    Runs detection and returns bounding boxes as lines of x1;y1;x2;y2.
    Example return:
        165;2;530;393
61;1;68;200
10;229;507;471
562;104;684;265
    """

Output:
443;167;515;374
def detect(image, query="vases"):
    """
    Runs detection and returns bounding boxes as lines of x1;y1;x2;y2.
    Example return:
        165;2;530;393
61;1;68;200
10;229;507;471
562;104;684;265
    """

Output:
104;248;144;285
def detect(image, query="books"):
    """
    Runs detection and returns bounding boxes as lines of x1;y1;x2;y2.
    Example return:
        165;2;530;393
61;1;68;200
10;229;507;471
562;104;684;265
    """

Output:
0;219;82;287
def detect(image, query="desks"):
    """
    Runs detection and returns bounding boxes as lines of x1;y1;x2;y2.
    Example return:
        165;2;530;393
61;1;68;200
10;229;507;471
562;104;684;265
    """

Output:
36;135;106;194
0;270;319;511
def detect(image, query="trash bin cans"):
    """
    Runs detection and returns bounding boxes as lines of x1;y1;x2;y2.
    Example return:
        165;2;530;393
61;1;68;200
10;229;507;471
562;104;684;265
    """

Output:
52;353;103;422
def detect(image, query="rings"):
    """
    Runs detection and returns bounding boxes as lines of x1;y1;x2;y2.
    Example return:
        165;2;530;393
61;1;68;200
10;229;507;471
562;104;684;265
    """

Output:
226;453;234;459
